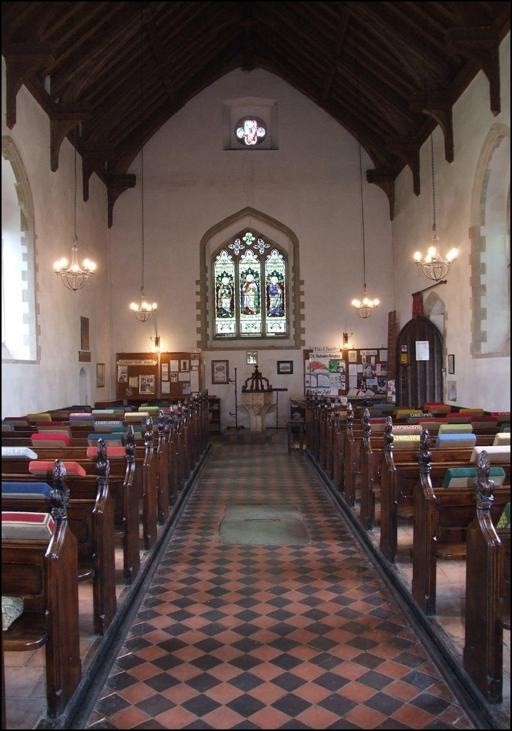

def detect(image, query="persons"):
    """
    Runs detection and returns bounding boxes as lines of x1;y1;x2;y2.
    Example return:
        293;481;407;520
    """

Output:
218;276;233;317
241;273;259;315
268;275;283;316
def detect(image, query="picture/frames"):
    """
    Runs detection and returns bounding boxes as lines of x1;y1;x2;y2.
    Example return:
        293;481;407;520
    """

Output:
277;360;293;374
448;354;454;373
211;360;229;384
96;363;105;387
246;350;257;365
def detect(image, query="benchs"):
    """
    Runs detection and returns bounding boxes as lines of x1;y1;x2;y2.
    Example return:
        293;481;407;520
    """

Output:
1;399;209;717
306;403;511;703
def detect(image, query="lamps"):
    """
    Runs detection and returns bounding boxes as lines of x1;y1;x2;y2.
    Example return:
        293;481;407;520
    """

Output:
54;147;97;290
128;146;158;321
351;143;380;318
413;111;459;281
